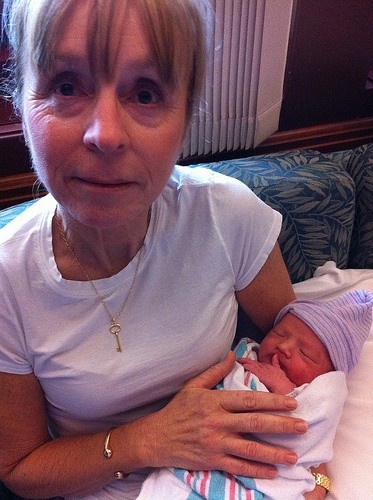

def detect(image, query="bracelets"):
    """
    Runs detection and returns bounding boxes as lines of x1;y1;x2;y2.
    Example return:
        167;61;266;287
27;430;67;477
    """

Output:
311;473;330;496
104;428;123;481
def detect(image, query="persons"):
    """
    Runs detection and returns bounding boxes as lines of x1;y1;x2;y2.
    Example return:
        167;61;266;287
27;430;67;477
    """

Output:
136;289;373;500
0;0;328;500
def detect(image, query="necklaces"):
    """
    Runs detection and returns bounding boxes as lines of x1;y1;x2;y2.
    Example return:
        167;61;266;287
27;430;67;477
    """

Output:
55;217;142;352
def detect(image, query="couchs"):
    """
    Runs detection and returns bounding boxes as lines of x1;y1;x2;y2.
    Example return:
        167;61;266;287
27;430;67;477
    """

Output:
0;119;373;285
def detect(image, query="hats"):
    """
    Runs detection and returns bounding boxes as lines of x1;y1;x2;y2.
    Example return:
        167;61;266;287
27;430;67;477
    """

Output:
273;290;373;377
